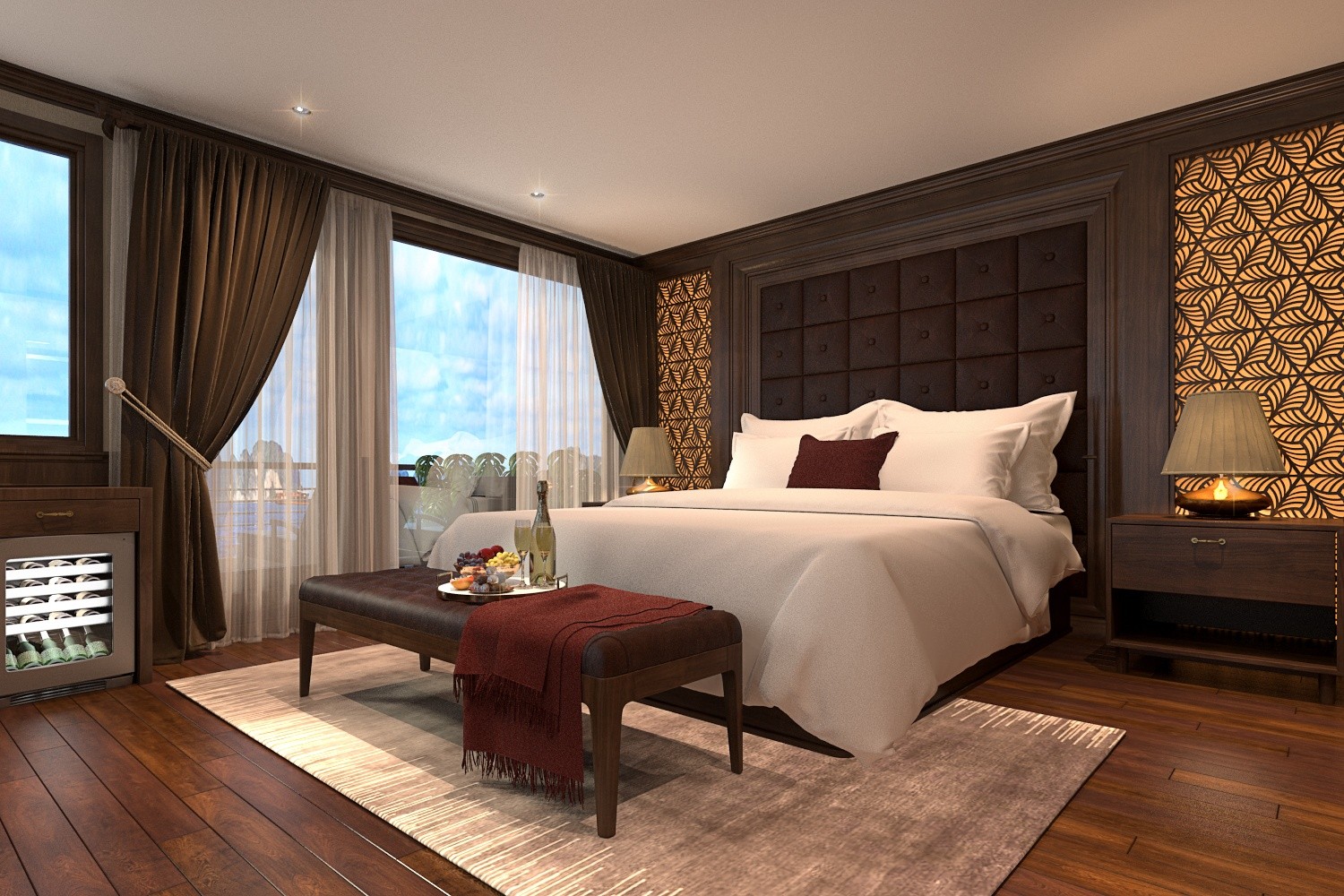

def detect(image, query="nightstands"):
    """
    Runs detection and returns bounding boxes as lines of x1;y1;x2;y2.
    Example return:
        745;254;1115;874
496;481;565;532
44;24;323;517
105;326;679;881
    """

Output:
1104;510;1344;709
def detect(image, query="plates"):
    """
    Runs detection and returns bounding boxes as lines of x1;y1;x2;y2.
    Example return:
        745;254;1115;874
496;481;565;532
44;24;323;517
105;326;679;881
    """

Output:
474;587;515;593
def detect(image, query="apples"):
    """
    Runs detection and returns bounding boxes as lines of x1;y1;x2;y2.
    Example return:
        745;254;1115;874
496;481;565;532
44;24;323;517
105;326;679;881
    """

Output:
477;548;495;563
450;575;474;590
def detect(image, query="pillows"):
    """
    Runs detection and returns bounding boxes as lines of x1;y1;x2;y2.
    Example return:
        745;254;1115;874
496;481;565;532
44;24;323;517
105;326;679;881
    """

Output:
739;396;886;440
869;420;1033;502
784;429;900;490
877;389;1077;515
721;422;857;489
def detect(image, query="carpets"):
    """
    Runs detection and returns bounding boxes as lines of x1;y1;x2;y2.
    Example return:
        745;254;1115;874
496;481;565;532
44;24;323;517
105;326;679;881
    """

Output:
161;643;1126;896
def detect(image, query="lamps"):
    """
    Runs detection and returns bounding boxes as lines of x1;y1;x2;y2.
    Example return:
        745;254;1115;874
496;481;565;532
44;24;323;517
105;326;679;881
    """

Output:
1159;389;1287;518
618;428;682;496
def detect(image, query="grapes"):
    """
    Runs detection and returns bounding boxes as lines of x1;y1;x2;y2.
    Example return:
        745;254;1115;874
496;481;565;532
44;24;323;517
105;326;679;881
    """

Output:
485;551;519;567
491;545;504;556
457;551;486;566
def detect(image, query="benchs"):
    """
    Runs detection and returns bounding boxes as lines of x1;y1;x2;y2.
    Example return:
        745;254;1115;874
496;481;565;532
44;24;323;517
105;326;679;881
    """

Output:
297;563;746;839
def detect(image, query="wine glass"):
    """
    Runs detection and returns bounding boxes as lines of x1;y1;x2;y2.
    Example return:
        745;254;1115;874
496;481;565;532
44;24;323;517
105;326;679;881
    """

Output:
536;522;553;589
514;517;533;589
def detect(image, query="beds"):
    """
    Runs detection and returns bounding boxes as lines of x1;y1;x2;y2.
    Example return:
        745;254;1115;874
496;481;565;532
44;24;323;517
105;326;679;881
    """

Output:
427;380;1087;766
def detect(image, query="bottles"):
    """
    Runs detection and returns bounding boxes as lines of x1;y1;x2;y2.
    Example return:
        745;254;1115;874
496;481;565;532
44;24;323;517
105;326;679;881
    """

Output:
529;481;556;586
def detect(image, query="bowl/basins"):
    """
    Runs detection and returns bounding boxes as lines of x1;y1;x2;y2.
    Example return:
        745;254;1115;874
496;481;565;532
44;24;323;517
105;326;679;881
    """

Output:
450;575;475;589
455;561;521;582
